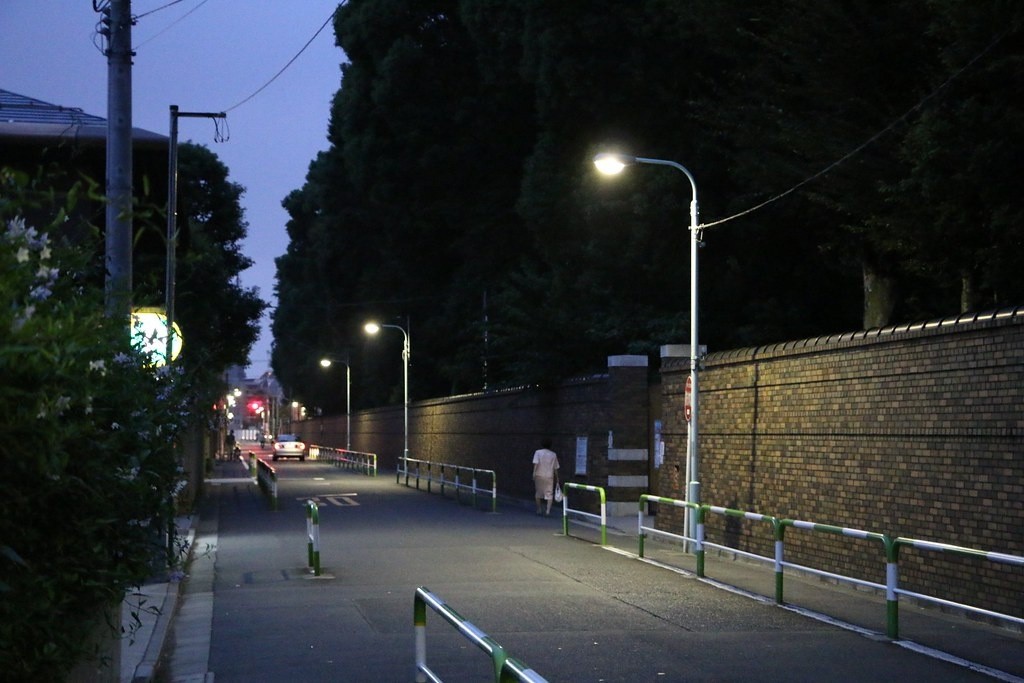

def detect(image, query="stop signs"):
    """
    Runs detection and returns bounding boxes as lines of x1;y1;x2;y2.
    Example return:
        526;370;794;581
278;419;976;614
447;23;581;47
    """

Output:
684;376;692;422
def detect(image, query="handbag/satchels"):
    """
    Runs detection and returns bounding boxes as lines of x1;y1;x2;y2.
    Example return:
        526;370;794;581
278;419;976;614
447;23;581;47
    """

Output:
554;486;563;502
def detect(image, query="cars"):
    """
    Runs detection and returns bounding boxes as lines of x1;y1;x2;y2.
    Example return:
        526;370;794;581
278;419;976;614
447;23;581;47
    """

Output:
272;434;306;462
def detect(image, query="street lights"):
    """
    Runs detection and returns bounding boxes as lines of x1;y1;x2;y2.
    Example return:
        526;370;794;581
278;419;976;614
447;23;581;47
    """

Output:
365;322;408;486
594;154;701;554
321;359;349;468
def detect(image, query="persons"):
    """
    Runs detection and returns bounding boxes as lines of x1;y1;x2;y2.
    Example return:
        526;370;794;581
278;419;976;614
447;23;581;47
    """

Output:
225;429;236;461
532;438;560;517
260;433;265;448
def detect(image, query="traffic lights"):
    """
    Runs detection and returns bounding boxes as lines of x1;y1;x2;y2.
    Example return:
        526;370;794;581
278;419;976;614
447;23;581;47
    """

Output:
249;399;260;411
